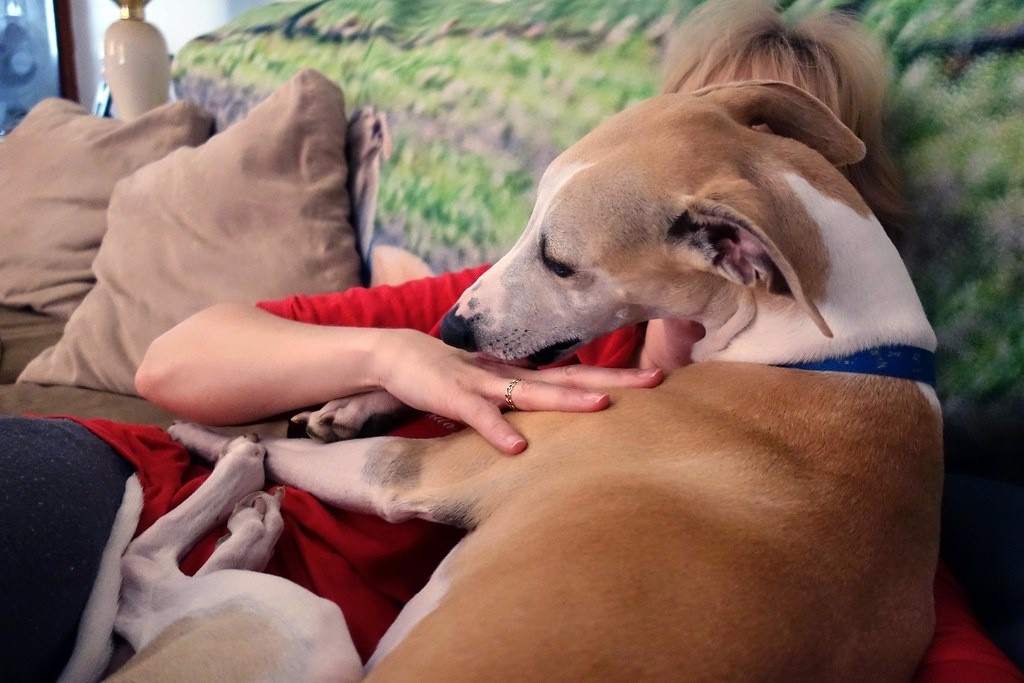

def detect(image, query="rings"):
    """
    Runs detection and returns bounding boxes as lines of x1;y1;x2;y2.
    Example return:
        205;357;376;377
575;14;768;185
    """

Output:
505;378;522;411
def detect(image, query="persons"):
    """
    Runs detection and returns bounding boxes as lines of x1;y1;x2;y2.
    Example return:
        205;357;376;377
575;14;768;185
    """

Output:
0;0;1024;683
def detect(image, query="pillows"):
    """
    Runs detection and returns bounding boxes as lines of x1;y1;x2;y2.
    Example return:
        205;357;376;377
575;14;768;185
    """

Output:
0;96;219;316
19;69;367;399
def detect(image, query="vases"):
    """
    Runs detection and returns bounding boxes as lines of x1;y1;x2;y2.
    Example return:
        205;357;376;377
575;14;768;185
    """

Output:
103;0;171;119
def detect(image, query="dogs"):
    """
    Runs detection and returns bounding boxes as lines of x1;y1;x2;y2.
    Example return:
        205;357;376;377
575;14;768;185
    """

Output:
61;80;947;683
